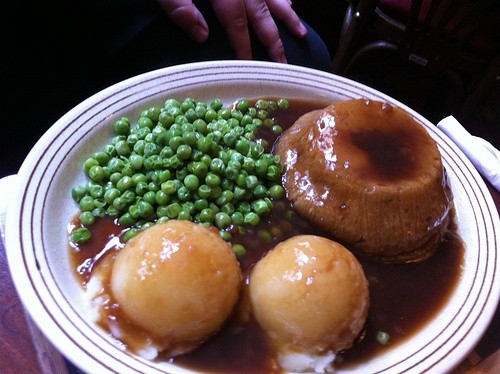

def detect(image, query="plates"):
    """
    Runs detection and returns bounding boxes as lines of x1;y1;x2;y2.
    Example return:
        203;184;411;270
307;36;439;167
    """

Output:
4;60;500;374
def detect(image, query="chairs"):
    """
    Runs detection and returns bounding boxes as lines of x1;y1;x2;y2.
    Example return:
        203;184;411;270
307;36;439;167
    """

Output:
328;0;500;117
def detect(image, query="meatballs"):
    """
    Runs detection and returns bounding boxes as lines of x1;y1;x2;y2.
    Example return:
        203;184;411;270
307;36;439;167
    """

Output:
248;235;371;357
110;218;242;345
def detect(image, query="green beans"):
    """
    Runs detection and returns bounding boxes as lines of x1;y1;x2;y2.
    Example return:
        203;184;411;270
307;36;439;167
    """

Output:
70;95;310;257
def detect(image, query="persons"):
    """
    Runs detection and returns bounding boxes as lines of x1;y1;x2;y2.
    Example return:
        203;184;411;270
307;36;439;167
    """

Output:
0;0;337;180
